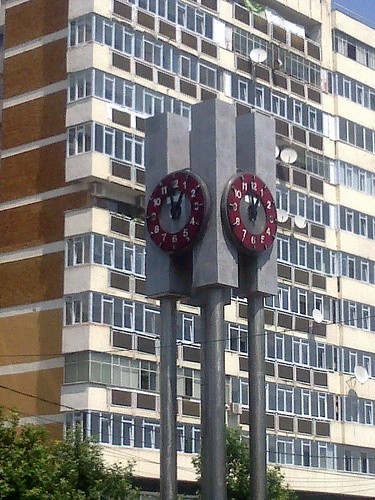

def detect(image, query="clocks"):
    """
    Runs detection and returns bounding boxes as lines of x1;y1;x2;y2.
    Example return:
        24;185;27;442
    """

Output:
146;170;212;251
221;171;279;256
